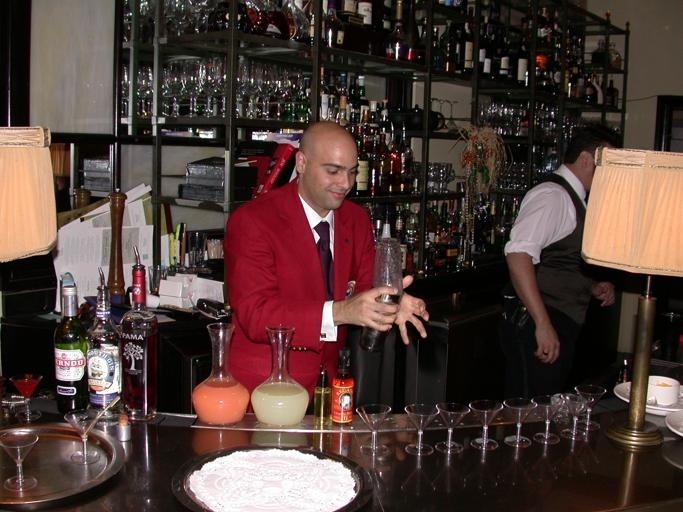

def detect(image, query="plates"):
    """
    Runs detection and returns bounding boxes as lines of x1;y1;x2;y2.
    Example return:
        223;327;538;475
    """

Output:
613;380;682;437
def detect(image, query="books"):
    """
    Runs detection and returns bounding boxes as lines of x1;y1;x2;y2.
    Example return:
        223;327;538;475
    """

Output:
46;183;176;316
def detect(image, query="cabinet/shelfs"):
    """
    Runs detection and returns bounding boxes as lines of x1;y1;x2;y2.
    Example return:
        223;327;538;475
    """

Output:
108;2;630;285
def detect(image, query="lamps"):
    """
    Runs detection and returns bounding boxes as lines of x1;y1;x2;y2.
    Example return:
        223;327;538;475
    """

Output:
581;144;683;449
0;123;60;265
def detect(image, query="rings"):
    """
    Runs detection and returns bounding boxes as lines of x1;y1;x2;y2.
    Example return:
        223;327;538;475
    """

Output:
541;351;549;356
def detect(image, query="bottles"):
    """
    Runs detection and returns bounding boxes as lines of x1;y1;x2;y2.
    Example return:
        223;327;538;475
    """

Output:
250;323;309;427
190;322;250;427
118;265;158;421
53;284;89;424
313;365;331;430
330;349;354;428
116;0;629;271
84;287;122;427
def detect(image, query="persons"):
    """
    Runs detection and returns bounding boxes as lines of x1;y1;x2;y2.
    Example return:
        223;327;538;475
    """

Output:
500;120;625;388
346;413;420;478
223;122;431;412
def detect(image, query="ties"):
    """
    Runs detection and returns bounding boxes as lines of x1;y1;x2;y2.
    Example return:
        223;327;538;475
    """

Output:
313;221;333;302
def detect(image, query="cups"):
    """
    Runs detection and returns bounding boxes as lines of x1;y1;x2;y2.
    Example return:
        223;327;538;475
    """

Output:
358;238;404;354
644;376;678;406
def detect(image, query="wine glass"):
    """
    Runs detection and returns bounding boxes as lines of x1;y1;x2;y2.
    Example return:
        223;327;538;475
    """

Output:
357;404;391;459
11;375;46;421
468;401;500;451
64;410;101;464
502;398;536;448
531;394;562;446
575;384;607;432
0;432;39;492
437;403;469;454
405;404;433;455
560;393;584;440
